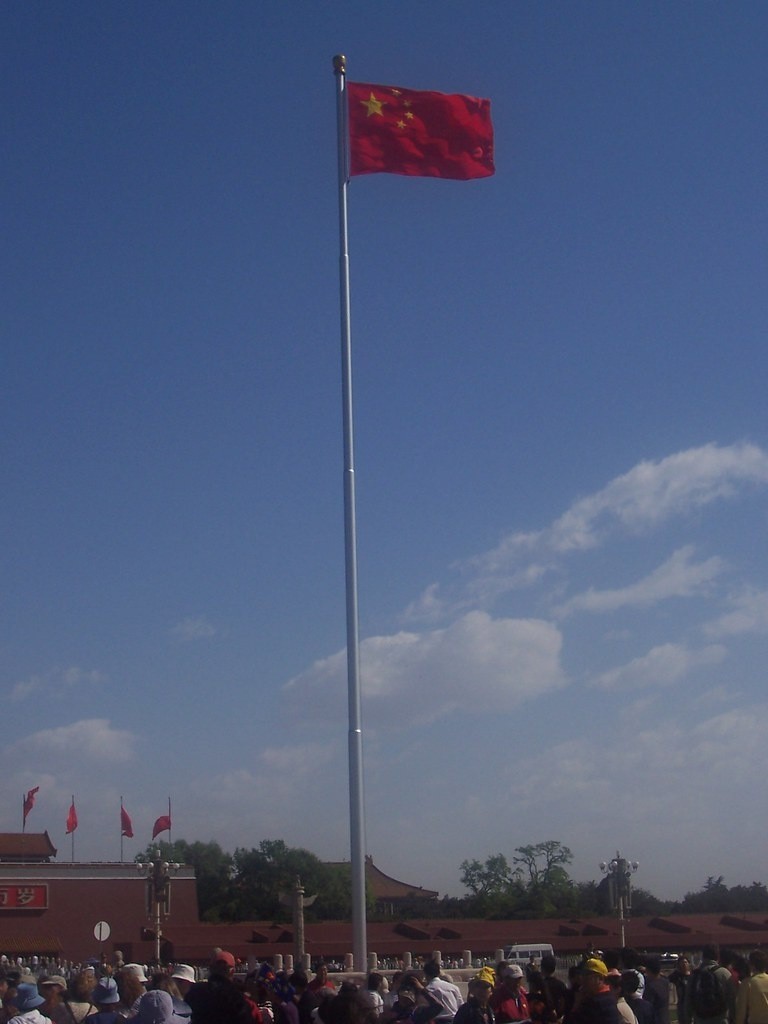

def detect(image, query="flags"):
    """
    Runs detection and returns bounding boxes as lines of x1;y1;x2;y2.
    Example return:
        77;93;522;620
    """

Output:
153;815;170;839
346;82;496;180
25;786;40;817
121;806;134;837
66;805;78;834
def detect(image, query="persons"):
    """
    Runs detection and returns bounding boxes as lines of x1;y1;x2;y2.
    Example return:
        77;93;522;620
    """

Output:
0;942;768;1024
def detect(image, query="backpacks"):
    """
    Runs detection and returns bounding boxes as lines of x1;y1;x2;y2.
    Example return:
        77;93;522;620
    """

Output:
690;965;722;1014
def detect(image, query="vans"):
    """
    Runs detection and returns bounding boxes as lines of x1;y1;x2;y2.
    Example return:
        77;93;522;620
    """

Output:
505;944;555;965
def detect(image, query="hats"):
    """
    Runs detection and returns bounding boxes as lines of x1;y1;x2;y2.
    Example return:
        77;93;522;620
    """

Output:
171;964;197;983
11;984;45;1010
120;963;149;982
608;969;622;976
502;964;523;978
42;975;66;989
23;976;38;983
483;966;495;974
131;990;191;1024
216;951;236;968
6;968;21;981
91;977;119;1004
584;959;607;976
468;970;496;987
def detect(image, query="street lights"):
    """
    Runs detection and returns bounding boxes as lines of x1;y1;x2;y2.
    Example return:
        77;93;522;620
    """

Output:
134;850;181;965
599;850;640;951
